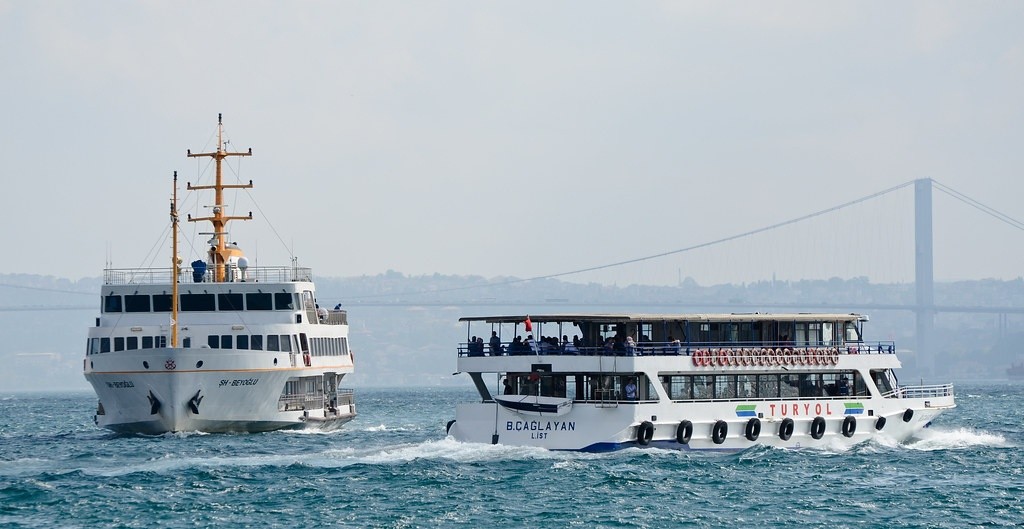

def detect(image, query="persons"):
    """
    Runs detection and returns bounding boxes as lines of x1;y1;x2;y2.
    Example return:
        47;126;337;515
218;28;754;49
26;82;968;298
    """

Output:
489;331;500;356
561;335;569;350
573;335;581;345
503;379;513;395
469;336;484;356
335;304;341;309
507;335;536;355
541;336;560;355
328;397;337;416
668;337;682;349
836;378;848;395
599;334;637;356
643;335;654;346
626;379;636;400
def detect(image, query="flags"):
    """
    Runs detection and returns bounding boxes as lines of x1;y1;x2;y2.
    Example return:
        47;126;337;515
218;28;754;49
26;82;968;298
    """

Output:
525;319;532;332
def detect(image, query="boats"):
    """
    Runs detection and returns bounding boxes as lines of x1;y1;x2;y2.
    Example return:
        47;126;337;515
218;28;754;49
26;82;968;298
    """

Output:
445;312;957;455
82;113;355;436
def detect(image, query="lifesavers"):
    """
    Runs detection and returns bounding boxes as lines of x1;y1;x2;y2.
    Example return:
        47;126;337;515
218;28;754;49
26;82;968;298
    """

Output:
745;417;762;441
903;408;914;423
875;416;887;430
842;415;857;438
637;421;655;446
712;419;728;445
692;347;840;366
811;416;826;440
779;417;795;441
676;419;693;445
